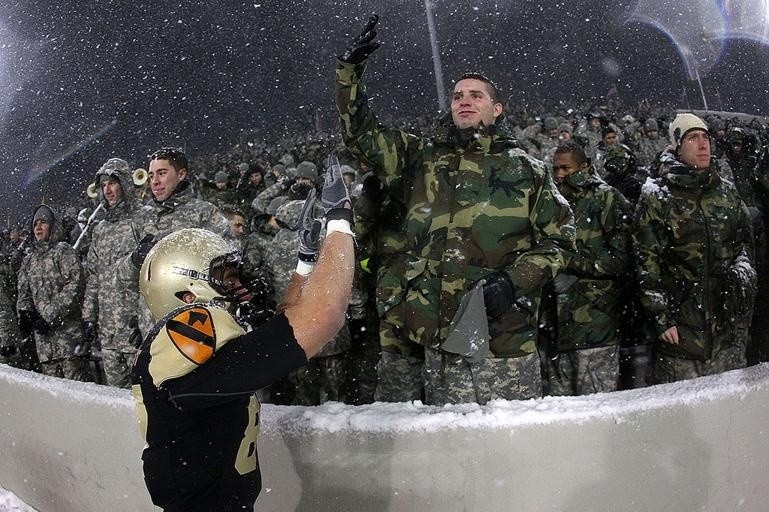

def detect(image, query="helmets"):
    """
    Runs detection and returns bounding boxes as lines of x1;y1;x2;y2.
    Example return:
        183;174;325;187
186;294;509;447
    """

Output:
138;228;242;320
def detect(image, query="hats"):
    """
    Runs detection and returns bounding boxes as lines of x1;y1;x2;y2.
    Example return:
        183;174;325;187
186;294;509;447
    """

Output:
668;113;711;152
33;206;53;228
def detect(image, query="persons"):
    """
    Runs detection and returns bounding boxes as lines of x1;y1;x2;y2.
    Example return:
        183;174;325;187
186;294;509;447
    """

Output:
1;129;382;405
502;99;768;397
383;109;438;134
132;153;360;512
334;13;576;405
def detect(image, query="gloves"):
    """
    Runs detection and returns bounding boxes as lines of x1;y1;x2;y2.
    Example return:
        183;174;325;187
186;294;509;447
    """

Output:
338;13;381;62
18;309;49;335
467;270;513;321
295;153;356;277
